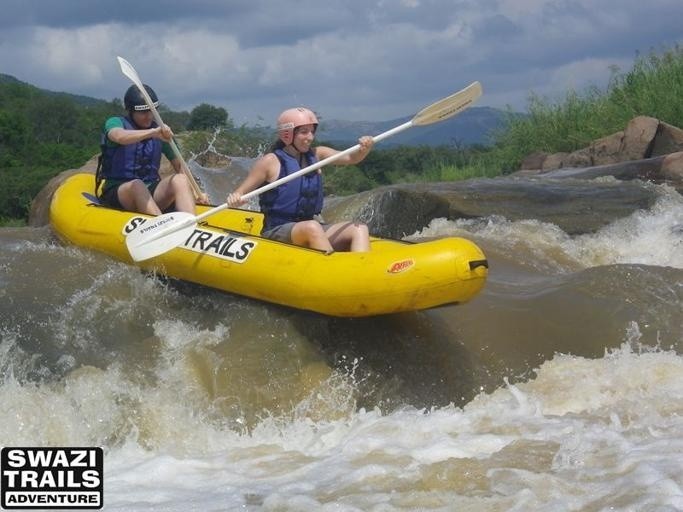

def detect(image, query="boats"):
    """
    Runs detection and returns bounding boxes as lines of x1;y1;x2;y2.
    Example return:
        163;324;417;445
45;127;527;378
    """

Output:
50;172;489;319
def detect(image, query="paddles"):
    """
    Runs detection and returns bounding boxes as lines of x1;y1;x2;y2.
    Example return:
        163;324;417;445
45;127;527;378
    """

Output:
124;80;483;263
117;56;202;202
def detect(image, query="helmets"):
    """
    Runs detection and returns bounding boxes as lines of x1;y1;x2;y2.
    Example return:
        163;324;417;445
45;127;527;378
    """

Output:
278;108;318;145
124;84;159;112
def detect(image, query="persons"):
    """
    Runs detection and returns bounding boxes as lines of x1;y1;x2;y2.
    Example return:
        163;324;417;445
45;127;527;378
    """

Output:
100;84;210;216
226;108;374;251
